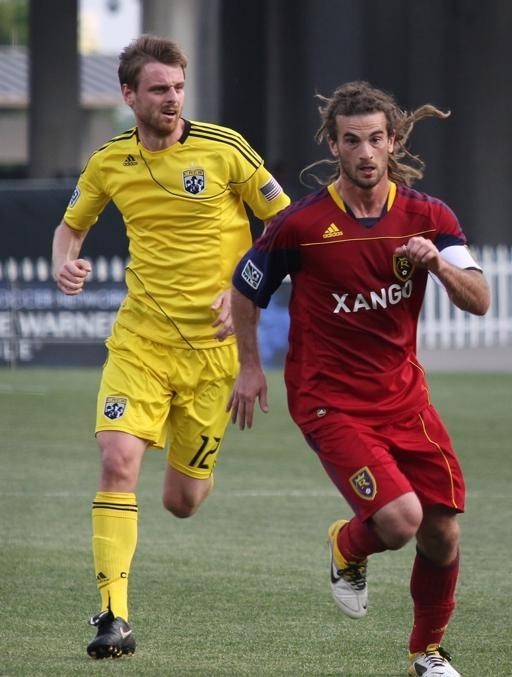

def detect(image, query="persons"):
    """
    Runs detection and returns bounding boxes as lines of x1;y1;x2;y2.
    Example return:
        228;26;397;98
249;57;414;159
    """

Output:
48;30;295;664
225;73;489;676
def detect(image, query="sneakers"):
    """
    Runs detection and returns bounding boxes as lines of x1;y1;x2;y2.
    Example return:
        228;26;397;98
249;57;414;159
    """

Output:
329;519;367;618
407;648;462;676
87;614;136;660
